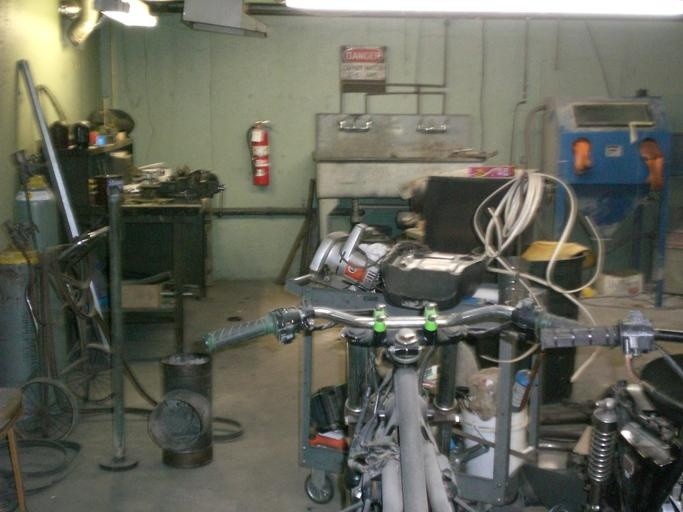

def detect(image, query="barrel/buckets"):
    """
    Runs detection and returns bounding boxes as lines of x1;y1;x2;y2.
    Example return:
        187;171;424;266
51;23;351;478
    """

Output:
466;238;590;407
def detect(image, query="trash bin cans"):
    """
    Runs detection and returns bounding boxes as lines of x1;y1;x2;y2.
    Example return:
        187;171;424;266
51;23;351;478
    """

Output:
499;253;585;403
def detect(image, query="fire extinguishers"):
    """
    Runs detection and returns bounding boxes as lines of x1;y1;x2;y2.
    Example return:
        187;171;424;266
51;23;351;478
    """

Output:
247;120;271;186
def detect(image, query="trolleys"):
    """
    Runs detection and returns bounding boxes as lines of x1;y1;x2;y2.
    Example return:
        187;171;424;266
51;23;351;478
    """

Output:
285;273;545;508
3;221;114;443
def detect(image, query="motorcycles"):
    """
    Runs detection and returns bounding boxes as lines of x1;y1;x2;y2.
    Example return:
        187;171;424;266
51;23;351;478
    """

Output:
540;309;681;511
204;306;589;511
